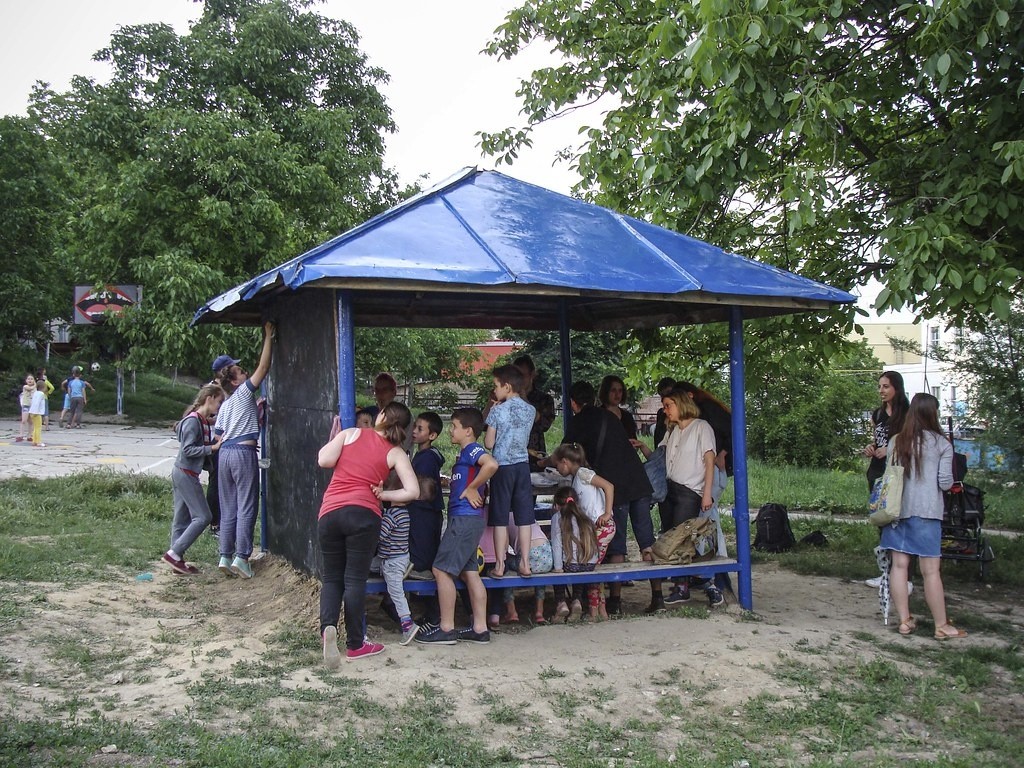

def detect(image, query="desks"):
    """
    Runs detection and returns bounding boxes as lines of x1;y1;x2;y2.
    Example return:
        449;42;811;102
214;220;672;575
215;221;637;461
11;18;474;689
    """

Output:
441;484;558;503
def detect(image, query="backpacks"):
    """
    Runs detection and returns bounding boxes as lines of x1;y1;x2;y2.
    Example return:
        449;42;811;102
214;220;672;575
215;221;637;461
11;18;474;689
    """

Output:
751;502;796;551
652;516;719;565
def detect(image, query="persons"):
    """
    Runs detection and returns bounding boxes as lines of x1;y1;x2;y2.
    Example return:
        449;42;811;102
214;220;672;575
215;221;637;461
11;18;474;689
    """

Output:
15;366;95;447
502;512;552;622
627;389;725;608
208;354;266;533
458;508;503;631
316;401;419;668
654;377;732;594
537;381;666;614
552;442;615;622
483;356;555;472
551;487;598;622
878;393;968;640
214;322;275;579
599;376;637;441
483;364;536;579
414;407;498;645
355;373;445;648
864;372;914;597
161;384;224;575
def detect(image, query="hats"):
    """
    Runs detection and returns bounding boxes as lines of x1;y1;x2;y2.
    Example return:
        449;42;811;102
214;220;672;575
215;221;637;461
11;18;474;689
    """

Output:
213;355;241;371
72;366;83;371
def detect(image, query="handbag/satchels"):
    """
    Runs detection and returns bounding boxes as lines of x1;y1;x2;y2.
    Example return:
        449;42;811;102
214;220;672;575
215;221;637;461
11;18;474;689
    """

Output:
870;467;904;526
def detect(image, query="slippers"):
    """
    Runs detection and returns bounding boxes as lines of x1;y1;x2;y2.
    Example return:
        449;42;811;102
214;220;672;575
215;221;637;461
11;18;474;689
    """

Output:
487;570;503;578
517;570;533;579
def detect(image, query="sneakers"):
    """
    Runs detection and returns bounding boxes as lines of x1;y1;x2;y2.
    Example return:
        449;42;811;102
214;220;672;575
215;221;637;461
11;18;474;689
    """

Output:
457;630;490;644
231;558;255;579
414;629;459;644
218;557;236;576
867;577;883;588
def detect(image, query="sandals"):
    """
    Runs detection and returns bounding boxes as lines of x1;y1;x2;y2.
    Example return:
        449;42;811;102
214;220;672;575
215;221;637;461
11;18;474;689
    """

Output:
934;621;967;642
900;617;919;634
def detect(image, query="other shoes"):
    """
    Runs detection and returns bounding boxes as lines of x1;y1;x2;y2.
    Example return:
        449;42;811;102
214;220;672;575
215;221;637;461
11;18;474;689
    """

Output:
321;626;342;669
489;622;499;630
707;587;723;607
509;612;519;623
17;436;47;447
416;617;441;638
667;592;692;603
399;625;419;644
346;637;385;661
607;595;622;617
533;608;546;623
571;600;584;624
551;603;569;625
645;597;666;612
162;553;191;575
210;528;220;537
42;419;81;431
172;566;202;576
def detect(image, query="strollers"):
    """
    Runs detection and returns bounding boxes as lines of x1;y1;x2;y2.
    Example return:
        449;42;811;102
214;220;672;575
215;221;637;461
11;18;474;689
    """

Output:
908;480;996;583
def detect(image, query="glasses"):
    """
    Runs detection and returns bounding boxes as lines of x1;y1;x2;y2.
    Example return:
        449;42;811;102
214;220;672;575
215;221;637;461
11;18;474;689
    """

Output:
663;402;675;409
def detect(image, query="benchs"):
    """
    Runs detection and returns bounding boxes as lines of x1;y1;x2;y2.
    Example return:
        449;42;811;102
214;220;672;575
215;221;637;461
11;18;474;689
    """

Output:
368;555;743;593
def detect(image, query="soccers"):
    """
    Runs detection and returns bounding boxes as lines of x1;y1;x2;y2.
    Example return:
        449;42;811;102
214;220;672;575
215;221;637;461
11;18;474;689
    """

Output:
477;545;485;573
91;362;100;372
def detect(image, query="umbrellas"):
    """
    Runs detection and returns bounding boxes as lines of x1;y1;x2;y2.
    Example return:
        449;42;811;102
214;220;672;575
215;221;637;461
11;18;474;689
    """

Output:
874;547;890;625
